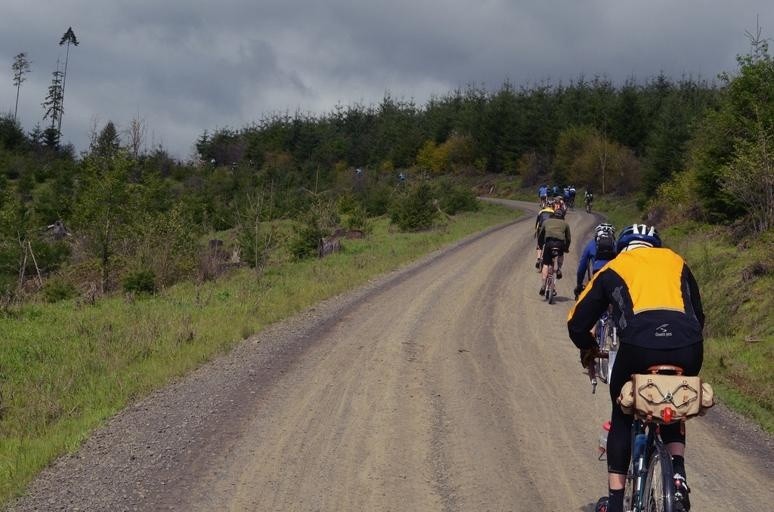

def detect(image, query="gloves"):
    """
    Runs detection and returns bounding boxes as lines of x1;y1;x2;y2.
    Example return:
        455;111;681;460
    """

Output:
580;343;598;368
574;287;581;296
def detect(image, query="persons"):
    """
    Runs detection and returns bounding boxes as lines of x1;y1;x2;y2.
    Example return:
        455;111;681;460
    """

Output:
572;220;622;357
533;181;596;298
565;222;705;512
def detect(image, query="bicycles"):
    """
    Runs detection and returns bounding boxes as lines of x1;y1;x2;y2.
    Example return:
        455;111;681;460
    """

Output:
596;313;618;384
546;248;559;304
593;351;678;512
540;198;592;213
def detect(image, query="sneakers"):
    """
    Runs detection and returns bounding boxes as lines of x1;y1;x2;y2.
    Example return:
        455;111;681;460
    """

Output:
540;288;545;296
557;273;562;279
596;497;610;512
536;262;539;268
674;478;691;511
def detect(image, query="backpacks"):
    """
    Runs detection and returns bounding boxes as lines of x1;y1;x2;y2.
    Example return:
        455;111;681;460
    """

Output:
597;233;615;260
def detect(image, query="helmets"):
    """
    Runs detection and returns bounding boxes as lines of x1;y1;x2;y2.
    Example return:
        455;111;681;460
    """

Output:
546;201;564;217
595;223;615;232
617;224;662;254
